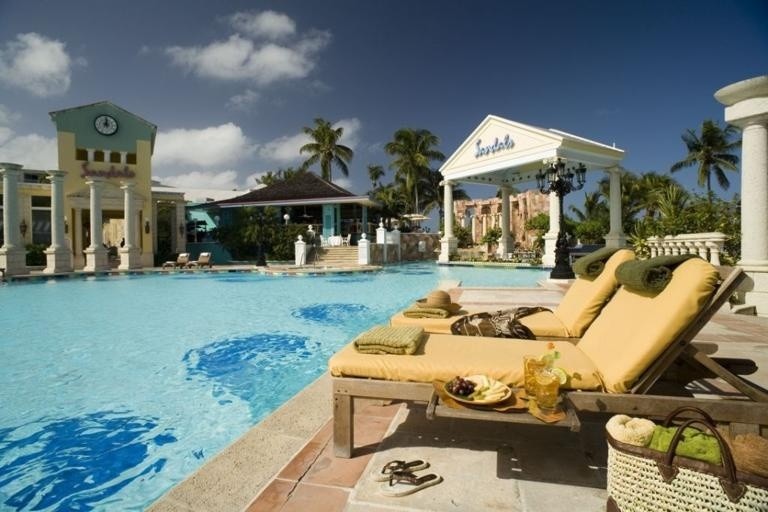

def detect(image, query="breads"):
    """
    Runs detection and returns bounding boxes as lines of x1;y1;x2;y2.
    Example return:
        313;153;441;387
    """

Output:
464;373;507;400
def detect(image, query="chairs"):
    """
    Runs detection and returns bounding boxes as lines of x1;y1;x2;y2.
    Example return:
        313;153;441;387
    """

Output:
326;241;768;463
161;250;215;271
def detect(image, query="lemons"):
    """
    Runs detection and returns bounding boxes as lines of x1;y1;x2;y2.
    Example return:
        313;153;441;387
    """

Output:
539;353;554;371
526;357;538;373
551;367;568;386
534;371;553;386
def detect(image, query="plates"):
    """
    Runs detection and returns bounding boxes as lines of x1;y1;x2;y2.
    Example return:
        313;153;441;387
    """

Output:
444;375;512;404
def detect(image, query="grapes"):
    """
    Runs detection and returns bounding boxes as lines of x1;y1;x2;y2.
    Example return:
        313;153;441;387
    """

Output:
449;376;478;397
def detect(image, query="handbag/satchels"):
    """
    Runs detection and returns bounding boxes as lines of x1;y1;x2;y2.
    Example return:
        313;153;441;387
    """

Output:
602;404;768;512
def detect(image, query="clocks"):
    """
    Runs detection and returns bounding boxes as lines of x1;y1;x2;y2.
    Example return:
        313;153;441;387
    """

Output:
94;114;119;136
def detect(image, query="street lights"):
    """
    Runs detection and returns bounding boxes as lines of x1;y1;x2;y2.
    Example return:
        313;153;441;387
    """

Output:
283;214;290;225
249;213;267;266
193;217;197;242
534;157;587;279
214;215;220;242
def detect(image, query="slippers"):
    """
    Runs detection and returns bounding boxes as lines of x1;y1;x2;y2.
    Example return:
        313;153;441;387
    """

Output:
377;469;443;498
368;459;428;484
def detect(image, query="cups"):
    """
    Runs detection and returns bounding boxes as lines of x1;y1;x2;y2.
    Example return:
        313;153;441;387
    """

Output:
523;357;547;400
535;373;560;415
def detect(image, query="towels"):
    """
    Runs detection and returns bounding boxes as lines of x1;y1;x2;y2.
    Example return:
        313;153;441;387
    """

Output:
707;426;768;483
